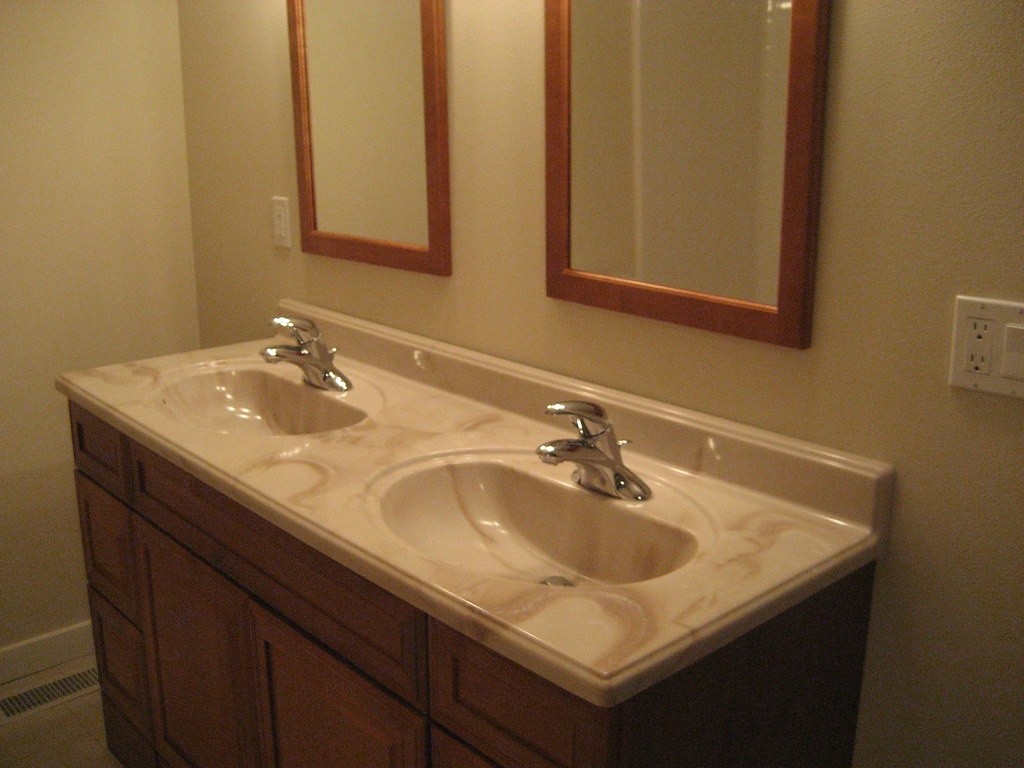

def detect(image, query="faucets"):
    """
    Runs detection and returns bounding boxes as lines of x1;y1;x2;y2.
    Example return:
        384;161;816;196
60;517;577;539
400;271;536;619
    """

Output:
535;399;653;503
259;314;354;393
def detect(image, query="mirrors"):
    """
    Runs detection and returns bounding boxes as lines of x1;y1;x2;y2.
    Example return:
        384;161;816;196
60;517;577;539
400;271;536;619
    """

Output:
287;0;451;275
545;0;833;350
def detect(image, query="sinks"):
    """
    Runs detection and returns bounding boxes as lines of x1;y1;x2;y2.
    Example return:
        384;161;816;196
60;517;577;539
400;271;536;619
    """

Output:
363;458;703;591
141;366;370;441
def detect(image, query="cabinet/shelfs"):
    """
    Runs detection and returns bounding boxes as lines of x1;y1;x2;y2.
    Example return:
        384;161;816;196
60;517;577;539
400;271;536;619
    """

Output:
66;398;872;768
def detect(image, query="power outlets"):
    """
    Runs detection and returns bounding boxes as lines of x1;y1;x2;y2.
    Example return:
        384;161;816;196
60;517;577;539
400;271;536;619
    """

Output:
272;196;290;248
949;295;1024;399
